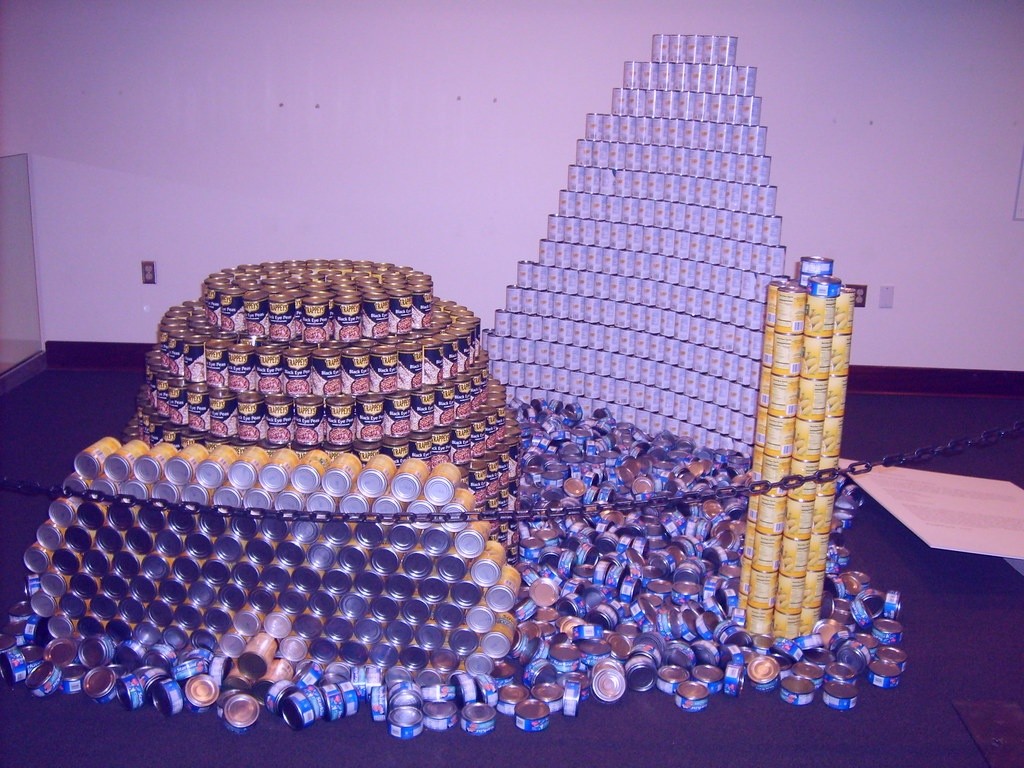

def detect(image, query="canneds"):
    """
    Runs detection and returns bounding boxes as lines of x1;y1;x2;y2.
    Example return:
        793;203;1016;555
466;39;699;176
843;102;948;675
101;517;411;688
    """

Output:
0;33;908;740
